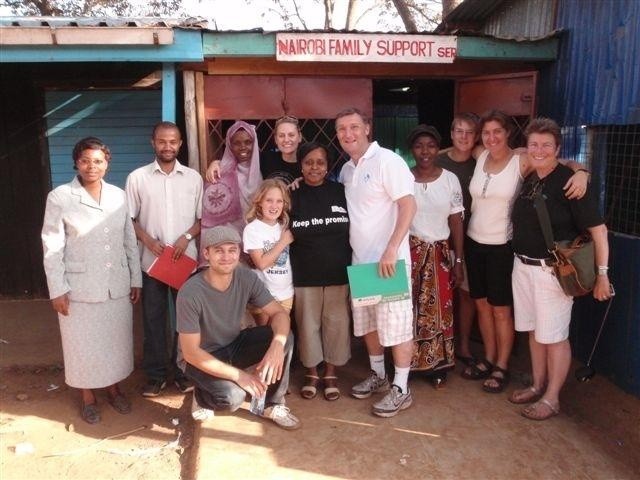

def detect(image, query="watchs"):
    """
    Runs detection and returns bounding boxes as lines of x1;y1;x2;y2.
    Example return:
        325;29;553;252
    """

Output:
184;233;192;241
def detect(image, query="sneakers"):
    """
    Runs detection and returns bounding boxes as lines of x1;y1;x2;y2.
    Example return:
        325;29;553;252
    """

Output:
142;376;215;422
81;400;99;423
108;393;129;414
352;369;389;398
261;404;301;430
372;385;412;418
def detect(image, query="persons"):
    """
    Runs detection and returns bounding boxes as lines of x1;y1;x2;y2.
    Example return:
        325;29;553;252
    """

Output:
202;107;612;421
42;136;143;423
124;121;203;396
175;226;302;430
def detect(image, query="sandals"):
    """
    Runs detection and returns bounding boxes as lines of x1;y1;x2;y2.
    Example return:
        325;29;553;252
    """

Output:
511;386;560;421
301;375;340;400
432;357;508;392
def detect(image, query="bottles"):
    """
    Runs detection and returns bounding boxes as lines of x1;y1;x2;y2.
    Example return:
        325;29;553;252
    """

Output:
250;372;265;416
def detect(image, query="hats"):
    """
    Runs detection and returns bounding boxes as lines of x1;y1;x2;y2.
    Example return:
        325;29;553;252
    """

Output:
407;125;442;145
205;226;242;248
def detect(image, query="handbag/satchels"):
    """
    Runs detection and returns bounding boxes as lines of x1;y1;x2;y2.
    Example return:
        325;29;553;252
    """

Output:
547;235;596;297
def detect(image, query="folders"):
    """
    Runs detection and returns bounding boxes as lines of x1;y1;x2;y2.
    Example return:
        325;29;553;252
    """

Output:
347;260;410;308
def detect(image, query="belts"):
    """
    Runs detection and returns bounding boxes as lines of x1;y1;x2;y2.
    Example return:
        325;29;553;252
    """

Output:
517;256;552;266
